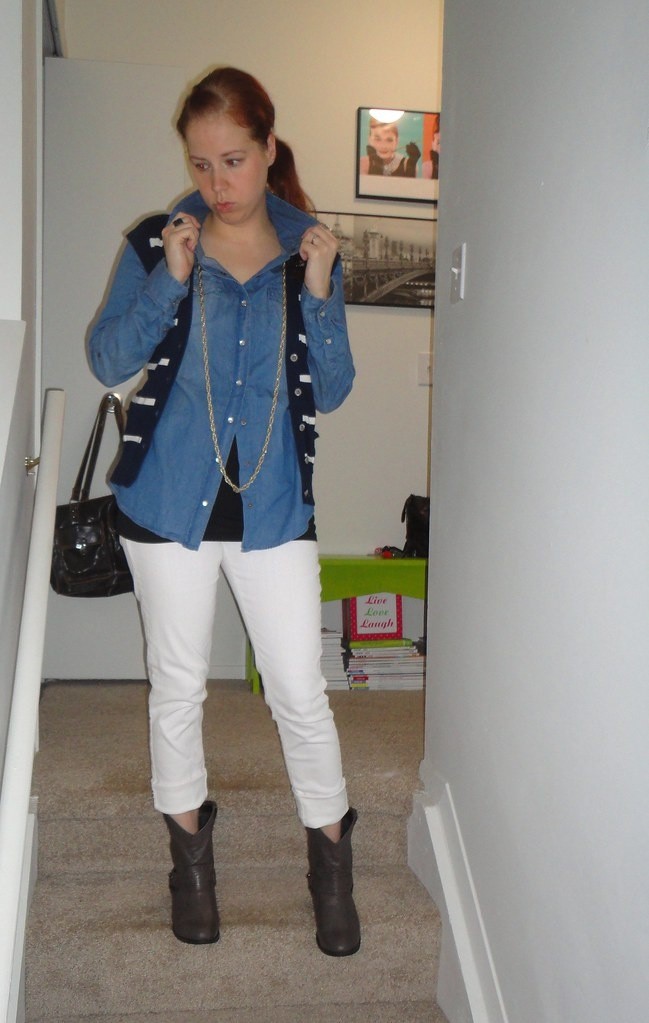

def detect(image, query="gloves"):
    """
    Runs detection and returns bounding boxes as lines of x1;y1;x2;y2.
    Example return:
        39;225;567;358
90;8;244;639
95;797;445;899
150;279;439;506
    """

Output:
430;150;439;179
366;144;377;175
406;141;421;178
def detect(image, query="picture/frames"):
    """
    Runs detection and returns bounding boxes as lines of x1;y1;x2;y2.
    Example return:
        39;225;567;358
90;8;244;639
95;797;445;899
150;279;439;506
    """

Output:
307;210;438;308
356;106;441;203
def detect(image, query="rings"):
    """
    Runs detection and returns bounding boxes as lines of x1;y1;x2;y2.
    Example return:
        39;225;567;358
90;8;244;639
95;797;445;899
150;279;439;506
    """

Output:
172;218;183;227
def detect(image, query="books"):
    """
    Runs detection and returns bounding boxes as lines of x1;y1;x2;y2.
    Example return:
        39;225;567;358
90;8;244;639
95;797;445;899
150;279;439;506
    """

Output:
318;631;426;691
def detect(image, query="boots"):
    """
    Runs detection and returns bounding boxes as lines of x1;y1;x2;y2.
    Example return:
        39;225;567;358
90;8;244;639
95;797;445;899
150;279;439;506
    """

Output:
163;800;220;944
305;806;361;957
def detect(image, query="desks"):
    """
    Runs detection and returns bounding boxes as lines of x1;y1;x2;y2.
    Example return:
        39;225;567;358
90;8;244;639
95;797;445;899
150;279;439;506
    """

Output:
245;556;428;694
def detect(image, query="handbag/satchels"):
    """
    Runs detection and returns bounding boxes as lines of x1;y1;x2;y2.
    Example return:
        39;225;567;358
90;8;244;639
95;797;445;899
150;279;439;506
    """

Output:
50;394;134;597
401;494;429;558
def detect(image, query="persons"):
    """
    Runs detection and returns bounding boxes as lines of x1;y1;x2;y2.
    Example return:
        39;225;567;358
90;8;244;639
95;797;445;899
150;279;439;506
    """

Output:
360;114;421;178
85;68;362;957
421;113;440;179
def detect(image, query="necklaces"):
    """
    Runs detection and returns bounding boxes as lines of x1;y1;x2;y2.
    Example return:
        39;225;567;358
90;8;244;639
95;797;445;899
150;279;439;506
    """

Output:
380;153;400;177
193;252;288;491
